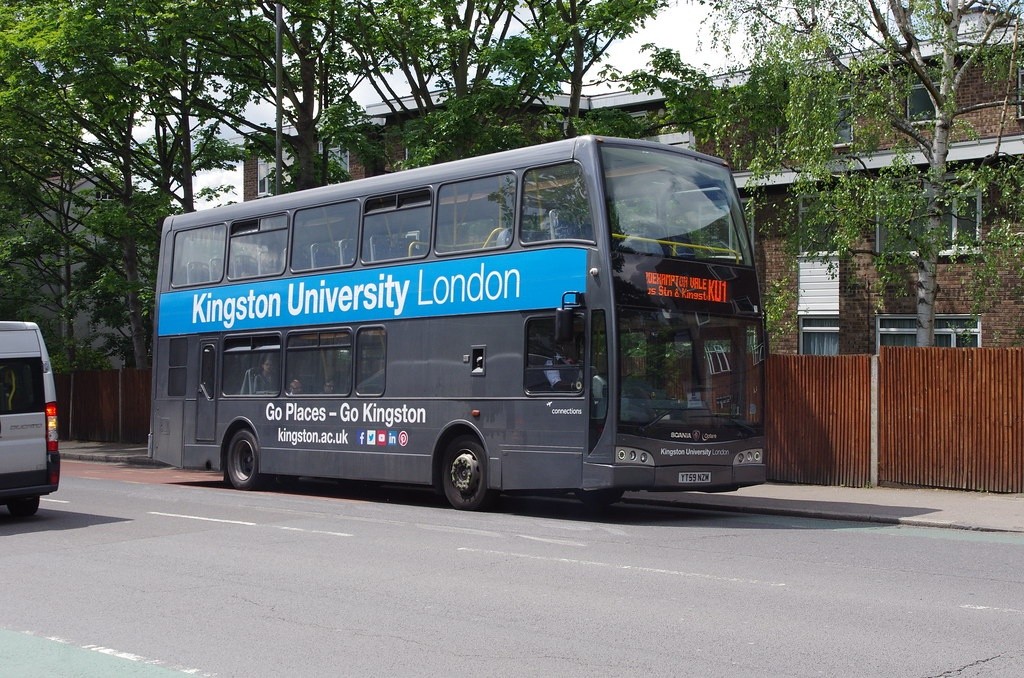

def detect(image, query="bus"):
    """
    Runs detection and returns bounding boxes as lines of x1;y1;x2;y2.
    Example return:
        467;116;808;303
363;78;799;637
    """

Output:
147;133;772;512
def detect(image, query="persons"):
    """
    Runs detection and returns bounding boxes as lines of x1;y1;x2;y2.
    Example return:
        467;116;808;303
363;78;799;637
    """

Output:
320;379;339;393
543;337;582;389
253;359;275;394
288;379;303;394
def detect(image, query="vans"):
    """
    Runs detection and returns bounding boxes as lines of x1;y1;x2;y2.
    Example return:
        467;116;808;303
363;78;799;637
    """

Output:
0;319;64;518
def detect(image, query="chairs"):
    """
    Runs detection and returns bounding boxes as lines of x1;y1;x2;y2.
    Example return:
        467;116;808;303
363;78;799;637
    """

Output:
248;366;265;395
186;207;583;284
